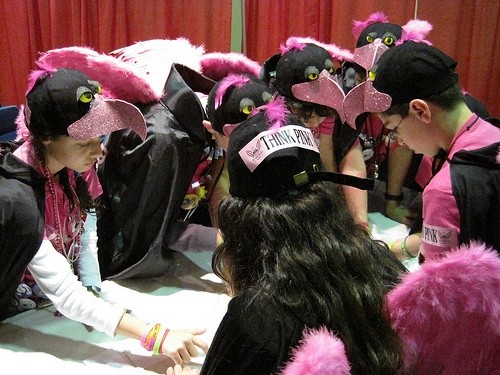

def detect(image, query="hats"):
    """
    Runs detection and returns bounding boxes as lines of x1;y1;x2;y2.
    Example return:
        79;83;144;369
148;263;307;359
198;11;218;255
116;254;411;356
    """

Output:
227;92;374;200
208;73;273;137
343;19;460;130
273;37;349;125
350;12;409;71
14;68;147;142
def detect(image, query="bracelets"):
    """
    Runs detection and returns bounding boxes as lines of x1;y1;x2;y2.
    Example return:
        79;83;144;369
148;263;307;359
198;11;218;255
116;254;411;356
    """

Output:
140;322;170;355
388;239;396;253
384;191;405;201
401;233;417;259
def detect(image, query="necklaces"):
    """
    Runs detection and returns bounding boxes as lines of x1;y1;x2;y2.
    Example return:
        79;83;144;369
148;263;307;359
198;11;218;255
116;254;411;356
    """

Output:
32;142;85;262
369;115;388;179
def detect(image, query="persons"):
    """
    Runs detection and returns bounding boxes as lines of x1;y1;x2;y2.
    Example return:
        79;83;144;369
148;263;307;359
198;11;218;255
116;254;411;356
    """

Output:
198;92;500;375
258;41;345;184
37;38;276;280
336;12;419;227
343;39;500;267
0;69;209;366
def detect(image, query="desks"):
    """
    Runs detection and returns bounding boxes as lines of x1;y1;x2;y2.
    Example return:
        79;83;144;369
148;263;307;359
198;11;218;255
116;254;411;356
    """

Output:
0;178;423;375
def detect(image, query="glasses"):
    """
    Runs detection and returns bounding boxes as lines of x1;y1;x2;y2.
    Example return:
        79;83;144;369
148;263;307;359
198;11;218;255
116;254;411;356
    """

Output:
387;114;404;140
